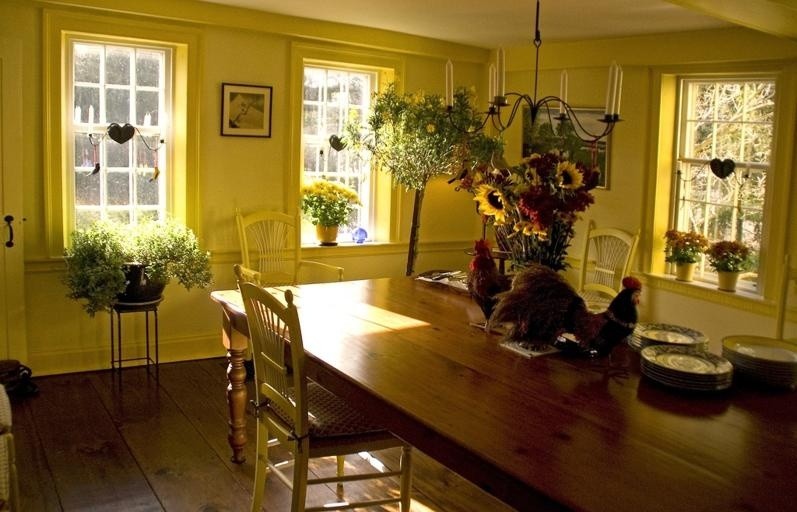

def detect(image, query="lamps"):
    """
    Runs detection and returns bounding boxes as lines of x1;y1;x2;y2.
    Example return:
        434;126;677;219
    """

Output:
445;1;634;143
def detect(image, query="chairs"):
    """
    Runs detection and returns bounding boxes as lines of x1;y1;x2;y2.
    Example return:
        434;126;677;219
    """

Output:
574;218;642;317
234;208;352;285
233;265;413;512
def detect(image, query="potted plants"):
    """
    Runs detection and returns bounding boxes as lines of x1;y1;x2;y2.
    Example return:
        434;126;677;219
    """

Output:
61;223;215;318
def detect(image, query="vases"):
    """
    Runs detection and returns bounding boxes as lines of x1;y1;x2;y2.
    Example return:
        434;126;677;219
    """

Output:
315;226;343;245
714;272;742;291
673;263;698;281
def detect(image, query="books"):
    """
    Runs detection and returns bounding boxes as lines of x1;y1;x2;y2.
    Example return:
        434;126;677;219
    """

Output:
500;340;562;360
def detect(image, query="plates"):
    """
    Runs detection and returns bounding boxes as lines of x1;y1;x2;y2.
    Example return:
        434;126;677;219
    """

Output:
639;344;733;391
720;334;796;389
631;321;709;352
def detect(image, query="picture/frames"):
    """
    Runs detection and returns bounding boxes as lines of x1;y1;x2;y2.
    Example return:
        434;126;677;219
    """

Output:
522;104;610;190
221;82;272;138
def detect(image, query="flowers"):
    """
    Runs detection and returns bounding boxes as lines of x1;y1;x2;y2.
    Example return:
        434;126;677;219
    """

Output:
298;180;362;223
344;81;510;276
706;242;756;270
660;229;708;262
460;153;597;265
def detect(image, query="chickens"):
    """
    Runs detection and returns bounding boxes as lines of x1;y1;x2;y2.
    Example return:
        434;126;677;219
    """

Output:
488;263;644;373
467;237;513;337
